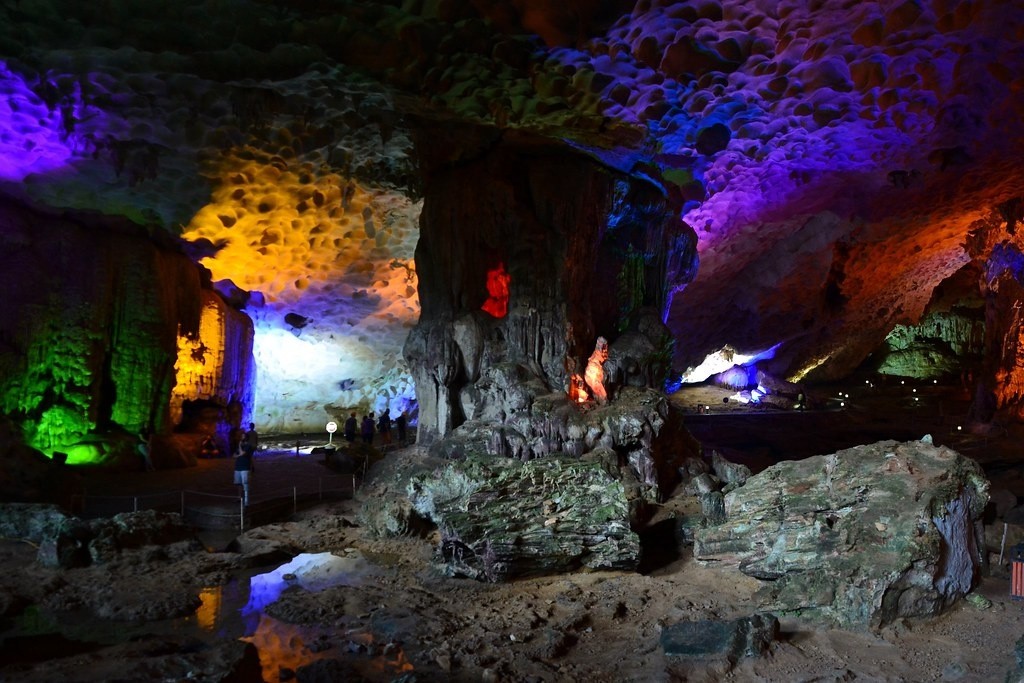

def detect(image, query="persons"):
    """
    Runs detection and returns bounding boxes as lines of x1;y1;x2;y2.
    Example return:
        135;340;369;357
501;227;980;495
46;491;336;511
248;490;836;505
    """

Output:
245;422;259;456
138;420;156;473
233;433;253;509
345;407;407;448
201;434;219;457
